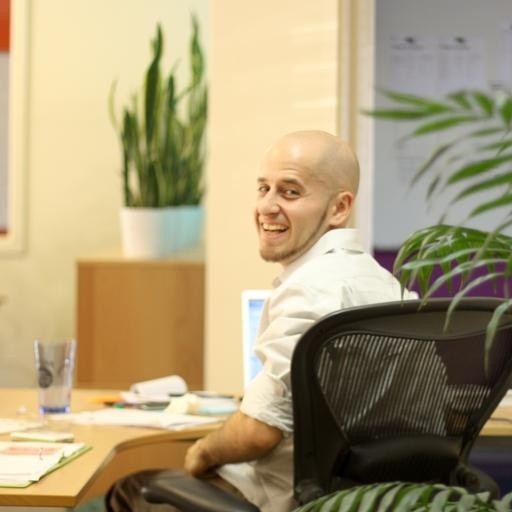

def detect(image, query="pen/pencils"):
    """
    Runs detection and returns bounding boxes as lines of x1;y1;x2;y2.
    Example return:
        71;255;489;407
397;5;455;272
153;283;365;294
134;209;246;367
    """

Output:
104;401;168;410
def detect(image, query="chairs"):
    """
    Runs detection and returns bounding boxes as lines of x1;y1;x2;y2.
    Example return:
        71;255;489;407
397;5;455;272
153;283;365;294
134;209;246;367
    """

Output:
141;294;512;512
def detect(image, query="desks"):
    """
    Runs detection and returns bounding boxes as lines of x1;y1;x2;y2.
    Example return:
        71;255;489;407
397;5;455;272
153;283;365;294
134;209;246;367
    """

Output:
0;387;241;510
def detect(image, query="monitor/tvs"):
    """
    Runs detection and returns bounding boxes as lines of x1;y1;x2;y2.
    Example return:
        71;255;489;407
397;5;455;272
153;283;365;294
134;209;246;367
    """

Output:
241;288;273;388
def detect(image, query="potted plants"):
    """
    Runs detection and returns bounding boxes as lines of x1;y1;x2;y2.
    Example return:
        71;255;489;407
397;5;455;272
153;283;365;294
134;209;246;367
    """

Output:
107;9;208;263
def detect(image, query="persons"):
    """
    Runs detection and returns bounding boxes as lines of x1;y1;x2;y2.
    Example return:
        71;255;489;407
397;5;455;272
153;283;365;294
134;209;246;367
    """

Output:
104;128;451;512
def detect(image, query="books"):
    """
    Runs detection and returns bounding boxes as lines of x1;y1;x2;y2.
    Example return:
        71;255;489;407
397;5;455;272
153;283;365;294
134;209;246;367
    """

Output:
1;435;95;488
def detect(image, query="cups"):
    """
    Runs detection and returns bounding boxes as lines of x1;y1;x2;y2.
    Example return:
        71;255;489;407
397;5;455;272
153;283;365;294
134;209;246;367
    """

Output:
32;337;78;415
